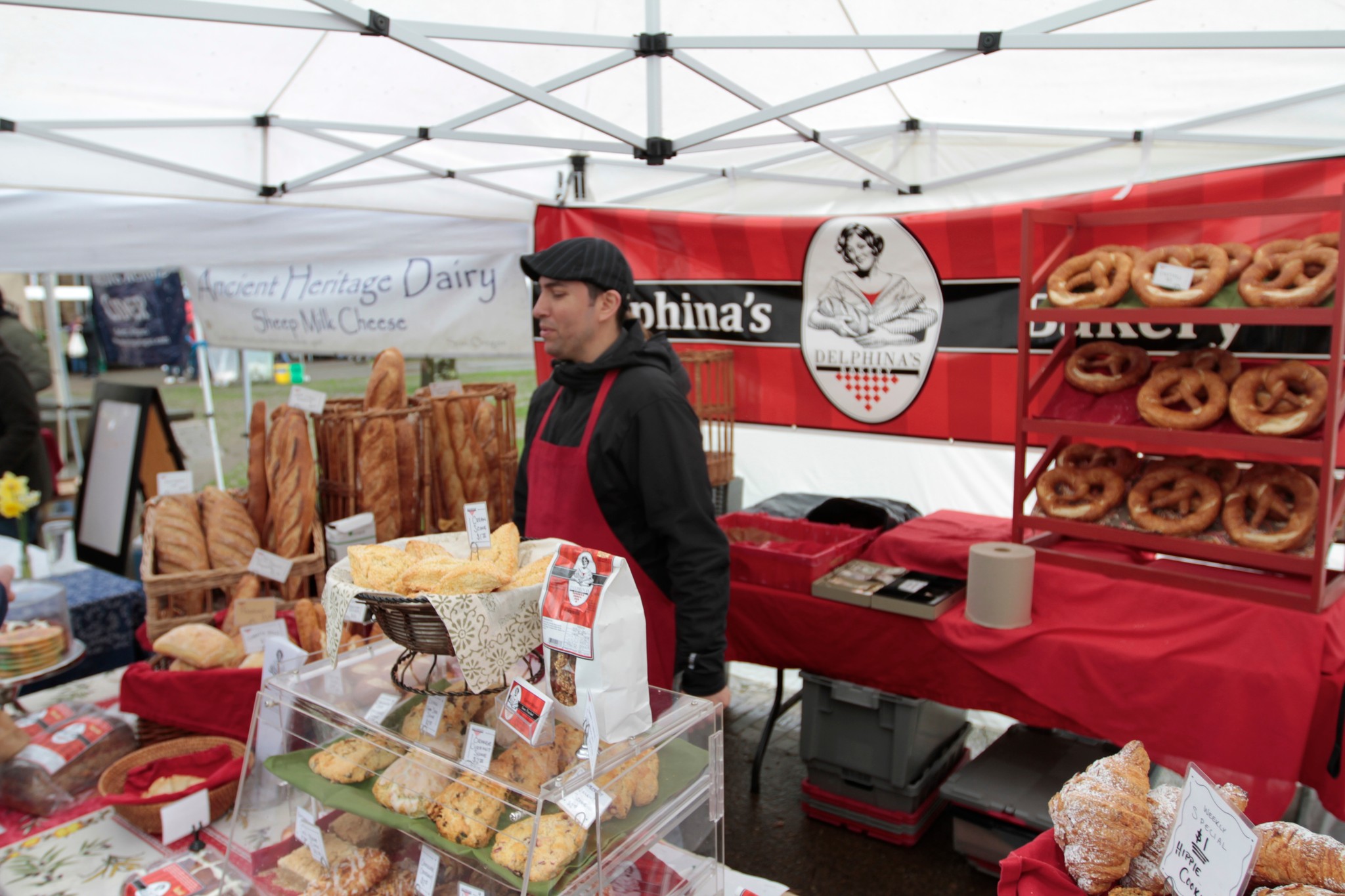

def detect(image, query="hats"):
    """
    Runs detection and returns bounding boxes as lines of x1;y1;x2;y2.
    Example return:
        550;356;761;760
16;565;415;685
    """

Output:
520;236;635;305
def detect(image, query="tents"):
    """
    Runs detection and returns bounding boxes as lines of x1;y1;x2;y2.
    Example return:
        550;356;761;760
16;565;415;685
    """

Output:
0;186;538;491
2;0;1345;216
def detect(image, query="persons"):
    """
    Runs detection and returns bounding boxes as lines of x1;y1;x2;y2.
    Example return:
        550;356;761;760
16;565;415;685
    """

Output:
0;566;15;628
509;237;730;721
0;331;54;547
0;288;52;391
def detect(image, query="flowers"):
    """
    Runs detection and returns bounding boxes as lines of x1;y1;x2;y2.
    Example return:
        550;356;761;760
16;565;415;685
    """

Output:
1;471;42;578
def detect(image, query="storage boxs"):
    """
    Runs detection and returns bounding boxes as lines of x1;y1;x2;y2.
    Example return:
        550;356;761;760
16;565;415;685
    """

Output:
799;748;971;846
136;496;328;645
804;720;977;810
714;510;871;595
798;670;969;786
938;722;1161;868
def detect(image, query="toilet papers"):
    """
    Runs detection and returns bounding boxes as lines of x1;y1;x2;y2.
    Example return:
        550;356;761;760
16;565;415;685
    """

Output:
965;543;1034;631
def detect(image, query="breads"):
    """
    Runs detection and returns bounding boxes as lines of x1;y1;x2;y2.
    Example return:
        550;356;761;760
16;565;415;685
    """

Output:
1046;739;1345;896
0;346;658;896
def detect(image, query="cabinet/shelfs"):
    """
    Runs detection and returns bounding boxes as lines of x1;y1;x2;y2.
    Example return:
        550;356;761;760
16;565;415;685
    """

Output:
215;638;729;896
1005;194;1345;614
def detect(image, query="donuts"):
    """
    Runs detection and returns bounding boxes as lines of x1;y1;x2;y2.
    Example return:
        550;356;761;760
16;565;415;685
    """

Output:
1038;232;1339;550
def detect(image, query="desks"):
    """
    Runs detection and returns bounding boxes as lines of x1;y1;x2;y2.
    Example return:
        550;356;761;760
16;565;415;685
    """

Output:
0;534;144;706
708;524;1345;802
0;659;801;896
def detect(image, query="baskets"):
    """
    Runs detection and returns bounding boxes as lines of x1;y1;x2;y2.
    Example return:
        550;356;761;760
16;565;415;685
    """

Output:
136;608;302;748
139;487;326;637
325;530;580;696
414;380;518;533
97;735;255;833
676;348;735;487
311;395;434;543
